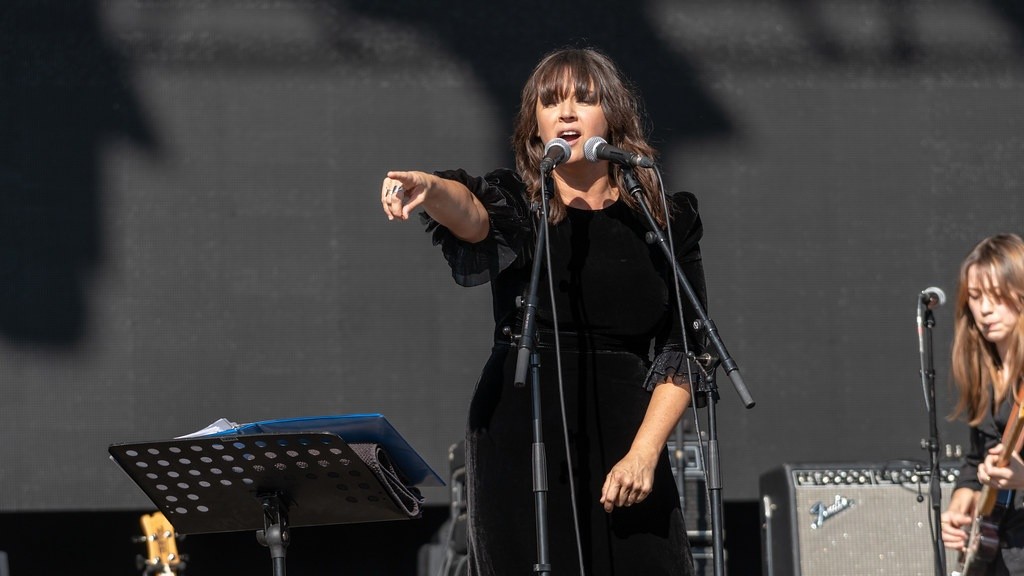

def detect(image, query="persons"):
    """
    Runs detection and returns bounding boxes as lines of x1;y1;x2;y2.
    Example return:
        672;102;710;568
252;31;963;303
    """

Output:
939;234;1024;576
380;35;711;576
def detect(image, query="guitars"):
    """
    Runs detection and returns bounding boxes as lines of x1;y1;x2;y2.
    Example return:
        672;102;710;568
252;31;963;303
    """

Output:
130;511;190;576
952;379;1024;576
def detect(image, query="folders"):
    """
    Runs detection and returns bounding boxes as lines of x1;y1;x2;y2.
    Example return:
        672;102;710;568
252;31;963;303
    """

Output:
163;415;447;491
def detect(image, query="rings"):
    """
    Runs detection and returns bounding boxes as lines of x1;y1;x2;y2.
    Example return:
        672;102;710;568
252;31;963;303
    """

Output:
385;189;393;198
392;185;405;194
987;476;992;485
941;521;950;530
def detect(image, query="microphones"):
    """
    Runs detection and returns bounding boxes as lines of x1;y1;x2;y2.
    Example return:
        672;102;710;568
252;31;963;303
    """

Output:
583;136;656;169
539;137;571;174
920;287;946;307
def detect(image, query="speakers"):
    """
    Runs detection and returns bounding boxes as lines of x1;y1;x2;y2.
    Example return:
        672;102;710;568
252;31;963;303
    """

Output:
759;459;968;576
689;547;728;576
666;431;725;544
